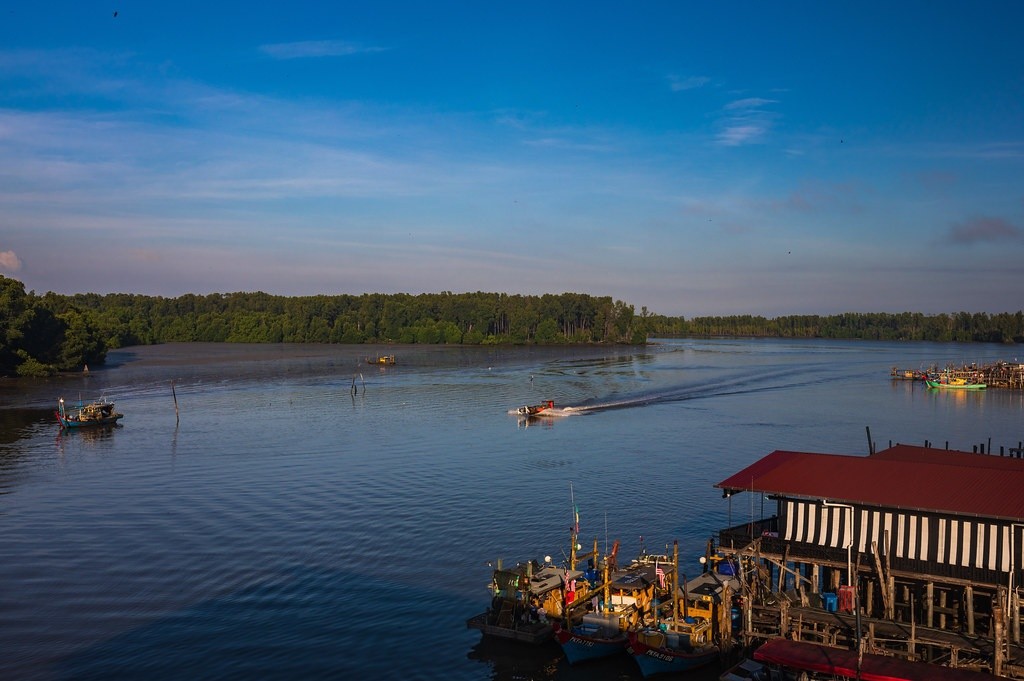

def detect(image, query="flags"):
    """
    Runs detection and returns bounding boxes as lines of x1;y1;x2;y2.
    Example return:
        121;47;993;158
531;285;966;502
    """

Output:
656;561;666;589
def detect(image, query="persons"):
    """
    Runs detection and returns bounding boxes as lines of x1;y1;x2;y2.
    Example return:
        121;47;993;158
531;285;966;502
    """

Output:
529;601;549;625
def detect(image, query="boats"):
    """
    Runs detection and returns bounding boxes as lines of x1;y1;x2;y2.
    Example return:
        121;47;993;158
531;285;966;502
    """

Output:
365;353;397;366
464;504;606;645
54;396;123;428
626;538;766;677
552;536;674;667
925;367;988;389
518;401;555;417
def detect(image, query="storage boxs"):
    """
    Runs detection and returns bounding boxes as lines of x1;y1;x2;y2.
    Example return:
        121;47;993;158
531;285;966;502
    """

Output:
579;623;601;636
